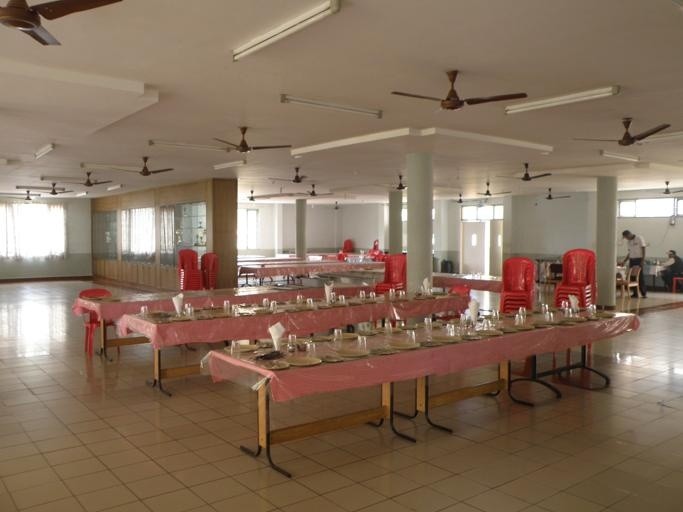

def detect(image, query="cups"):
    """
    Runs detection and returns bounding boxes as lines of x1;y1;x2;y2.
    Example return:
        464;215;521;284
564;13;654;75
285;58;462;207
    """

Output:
344;255;374;264
540;300;568;314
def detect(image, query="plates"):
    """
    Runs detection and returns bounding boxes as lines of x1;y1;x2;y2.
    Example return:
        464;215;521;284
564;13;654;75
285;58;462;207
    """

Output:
225;305;617;369
133;291;456;325
84;296;120;303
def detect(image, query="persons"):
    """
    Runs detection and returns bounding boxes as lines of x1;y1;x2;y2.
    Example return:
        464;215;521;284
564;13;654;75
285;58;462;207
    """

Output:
661;250;683;291
622;230;647;298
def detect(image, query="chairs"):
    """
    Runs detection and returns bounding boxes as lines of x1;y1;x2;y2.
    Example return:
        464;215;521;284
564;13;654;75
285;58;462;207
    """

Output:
343;240;353;252
672;277;683;293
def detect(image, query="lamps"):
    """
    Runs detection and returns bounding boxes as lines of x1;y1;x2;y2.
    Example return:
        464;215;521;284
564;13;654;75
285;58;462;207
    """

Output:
505;84;621;114
231;0;343;62
279;92;384;119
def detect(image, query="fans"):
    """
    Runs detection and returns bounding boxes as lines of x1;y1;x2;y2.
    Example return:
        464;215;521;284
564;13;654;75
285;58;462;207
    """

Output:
0;0;124;46
390;70;528;115
